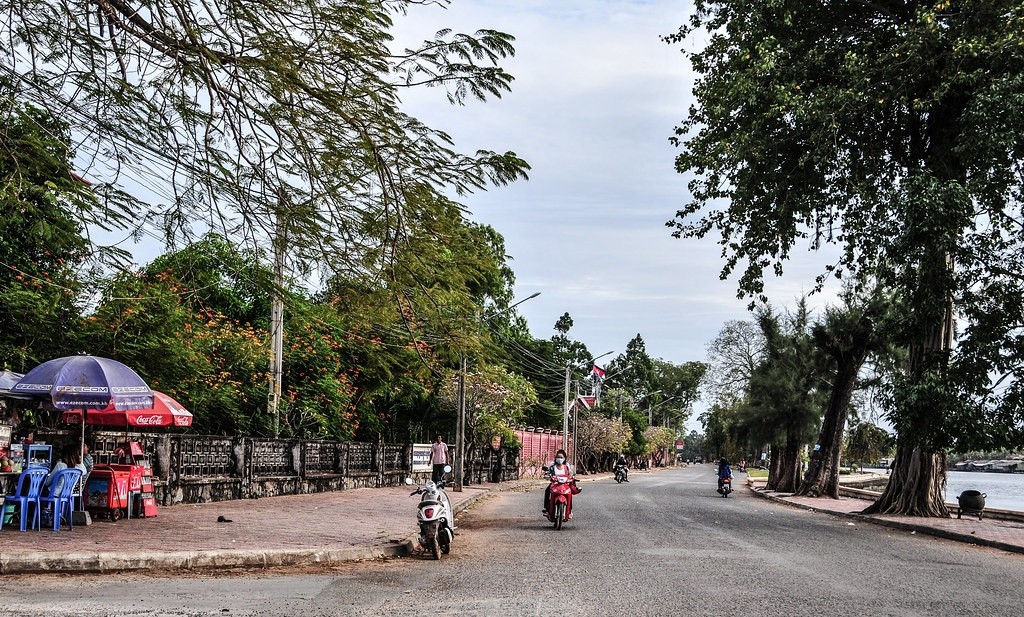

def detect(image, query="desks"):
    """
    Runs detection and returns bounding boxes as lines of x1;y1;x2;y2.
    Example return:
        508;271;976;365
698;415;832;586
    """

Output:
0;470;48;494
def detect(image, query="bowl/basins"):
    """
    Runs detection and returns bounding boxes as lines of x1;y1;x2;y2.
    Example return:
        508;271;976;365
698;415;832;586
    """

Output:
34;440;45;445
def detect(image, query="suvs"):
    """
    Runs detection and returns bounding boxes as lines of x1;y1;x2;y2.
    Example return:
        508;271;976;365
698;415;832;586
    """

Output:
693;454;705;465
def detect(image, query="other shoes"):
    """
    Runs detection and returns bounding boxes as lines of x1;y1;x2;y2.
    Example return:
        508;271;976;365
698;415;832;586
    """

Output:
627;480;629;482
569;514;573;519
542;508;546;512
718;488;721;490
730;487;733;490
543;512;549;515
614;477;617;480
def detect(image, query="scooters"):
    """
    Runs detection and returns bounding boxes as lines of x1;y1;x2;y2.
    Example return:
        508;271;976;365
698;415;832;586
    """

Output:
406;465;455;560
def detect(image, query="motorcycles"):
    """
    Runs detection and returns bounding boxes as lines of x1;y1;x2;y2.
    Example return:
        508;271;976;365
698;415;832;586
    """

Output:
614;460;626;483
714;469;735;498
542;466;580;529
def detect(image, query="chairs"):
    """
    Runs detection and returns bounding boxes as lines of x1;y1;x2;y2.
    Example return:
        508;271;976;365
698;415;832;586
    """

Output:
32;468;83;532
0;467;50;532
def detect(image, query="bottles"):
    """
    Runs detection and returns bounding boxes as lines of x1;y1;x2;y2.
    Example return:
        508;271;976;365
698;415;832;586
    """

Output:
13;455;23;473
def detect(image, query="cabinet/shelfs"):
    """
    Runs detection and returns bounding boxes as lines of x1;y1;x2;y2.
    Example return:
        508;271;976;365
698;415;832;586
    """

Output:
22;444;52;472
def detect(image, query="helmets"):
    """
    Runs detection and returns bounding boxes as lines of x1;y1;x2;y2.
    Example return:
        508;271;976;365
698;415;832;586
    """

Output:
569;482;582;495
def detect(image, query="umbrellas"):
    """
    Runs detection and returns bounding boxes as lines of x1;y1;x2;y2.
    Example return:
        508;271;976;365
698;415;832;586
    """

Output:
8;354;155;512
0;368;34;424
63;389;193;464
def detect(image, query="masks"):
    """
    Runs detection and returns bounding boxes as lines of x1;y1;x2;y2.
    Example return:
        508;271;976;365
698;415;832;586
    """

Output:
556;457;564;464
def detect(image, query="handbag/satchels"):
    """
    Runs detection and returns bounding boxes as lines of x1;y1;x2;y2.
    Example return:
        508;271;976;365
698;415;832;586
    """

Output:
741;468;744;471
730;473;734;479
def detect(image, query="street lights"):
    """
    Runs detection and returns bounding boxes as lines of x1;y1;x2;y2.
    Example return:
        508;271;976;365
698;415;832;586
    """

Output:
571;366;632;472
563;351;614;455
618;390;682;470
268;162;394;439
452;291;541;491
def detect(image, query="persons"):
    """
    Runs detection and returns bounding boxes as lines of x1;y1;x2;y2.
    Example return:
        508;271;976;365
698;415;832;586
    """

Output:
614;455;629;482
428;435;449;489
717;457;734;494
24;408;44;429
738;458;748;472
43;441;94;512
542;449;574;522
680;456;696;465
